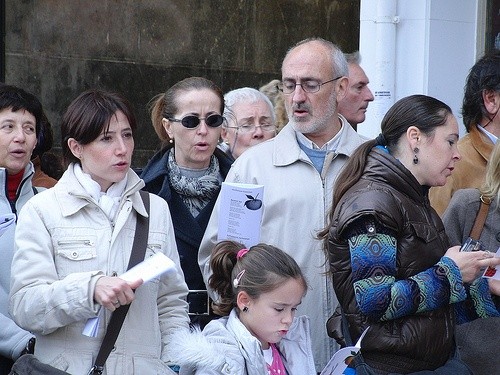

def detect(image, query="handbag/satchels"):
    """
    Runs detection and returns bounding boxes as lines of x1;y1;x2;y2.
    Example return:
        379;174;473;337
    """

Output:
317;330;370;375
8;339;110;375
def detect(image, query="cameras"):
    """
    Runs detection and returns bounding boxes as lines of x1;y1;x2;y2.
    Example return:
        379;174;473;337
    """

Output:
460;238;485;253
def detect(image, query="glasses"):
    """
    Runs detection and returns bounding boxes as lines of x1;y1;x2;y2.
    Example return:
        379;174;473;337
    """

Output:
227;122;275;133
168;114;227;129
275;77;340;95
